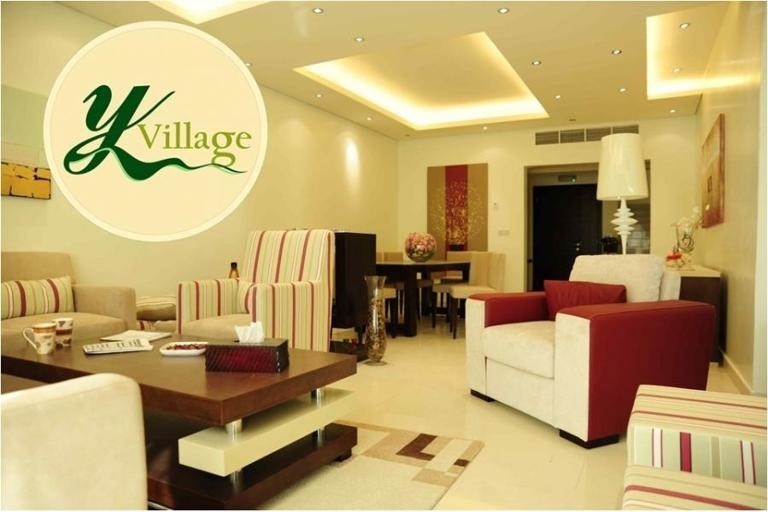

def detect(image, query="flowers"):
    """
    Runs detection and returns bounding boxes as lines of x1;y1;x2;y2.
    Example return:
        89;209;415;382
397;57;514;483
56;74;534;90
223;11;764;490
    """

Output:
681;206;702;224
403;230;436;256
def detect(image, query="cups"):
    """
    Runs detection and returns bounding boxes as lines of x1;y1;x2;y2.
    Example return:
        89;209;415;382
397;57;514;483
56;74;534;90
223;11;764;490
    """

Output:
23;321;57;354
54;317;73;349
665;252;692;271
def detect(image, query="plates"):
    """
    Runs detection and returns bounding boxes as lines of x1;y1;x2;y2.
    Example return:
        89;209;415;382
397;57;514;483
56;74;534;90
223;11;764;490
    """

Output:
160;342;207;356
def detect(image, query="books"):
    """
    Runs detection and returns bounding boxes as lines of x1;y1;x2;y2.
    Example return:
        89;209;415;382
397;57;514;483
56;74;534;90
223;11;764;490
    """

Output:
81;337;155;355
100;328;174;342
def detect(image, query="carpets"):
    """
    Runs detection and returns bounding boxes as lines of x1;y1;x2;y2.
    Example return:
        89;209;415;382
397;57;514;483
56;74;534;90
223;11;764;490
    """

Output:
255;419;484;510
330;339;370;362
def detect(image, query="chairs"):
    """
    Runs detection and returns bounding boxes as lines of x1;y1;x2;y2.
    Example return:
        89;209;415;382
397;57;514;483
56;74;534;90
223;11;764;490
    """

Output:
175;230;336;353
465;253;715;449
1;371;147;511
621;379;766;510
370;247;506;342
1;252;136;351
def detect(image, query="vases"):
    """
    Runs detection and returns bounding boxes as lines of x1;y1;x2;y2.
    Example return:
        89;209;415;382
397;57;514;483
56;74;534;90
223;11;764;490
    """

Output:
362;271;388;367
670;223;703;270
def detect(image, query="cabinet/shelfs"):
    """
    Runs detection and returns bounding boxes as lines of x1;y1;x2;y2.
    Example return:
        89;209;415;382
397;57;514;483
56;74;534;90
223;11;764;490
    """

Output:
664;265;728;368
331;229;380;350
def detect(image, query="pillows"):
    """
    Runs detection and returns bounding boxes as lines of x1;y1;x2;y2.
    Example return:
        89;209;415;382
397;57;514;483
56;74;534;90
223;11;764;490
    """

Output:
1;275;77;321
544;277;627;322
236;279;261;315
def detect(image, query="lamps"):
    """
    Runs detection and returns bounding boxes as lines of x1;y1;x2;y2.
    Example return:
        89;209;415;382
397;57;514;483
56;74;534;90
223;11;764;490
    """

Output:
595;134;649;254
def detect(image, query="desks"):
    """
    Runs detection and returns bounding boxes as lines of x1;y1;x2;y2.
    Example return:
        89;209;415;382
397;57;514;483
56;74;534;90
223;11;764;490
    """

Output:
136;294;175;333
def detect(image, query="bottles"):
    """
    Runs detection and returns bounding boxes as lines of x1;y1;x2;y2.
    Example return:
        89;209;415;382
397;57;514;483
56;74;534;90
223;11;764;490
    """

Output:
227;262;240;280
